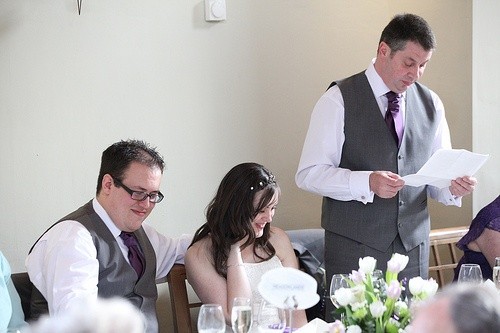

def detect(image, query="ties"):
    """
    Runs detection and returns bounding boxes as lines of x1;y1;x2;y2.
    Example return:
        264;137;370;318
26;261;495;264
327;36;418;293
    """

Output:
121;230;145;282
385;91;404;149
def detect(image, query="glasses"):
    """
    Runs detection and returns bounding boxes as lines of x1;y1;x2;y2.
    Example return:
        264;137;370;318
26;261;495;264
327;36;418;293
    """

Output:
115;180;164;203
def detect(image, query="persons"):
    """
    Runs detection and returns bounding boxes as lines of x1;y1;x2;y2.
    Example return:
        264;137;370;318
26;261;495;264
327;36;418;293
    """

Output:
184;163;308;333
411;279;500;333
294;12;478;323
452;194;500;283
24;139;194;333
0;249;32;333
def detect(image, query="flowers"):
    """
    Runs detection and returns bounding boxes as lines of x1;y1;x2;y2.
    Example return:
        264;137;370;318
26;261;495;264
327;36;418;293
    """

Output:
332;253;438;333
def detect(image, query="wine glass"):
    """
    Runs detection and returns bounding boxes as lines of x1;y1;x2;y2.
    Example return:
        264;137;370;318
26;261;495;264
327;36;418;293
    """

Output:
330;274;354;324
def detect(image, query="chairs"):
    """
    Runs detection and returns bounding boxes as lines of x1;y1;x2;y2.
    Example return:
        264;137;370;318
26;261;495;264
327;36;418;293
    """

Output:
430;225;469;287
167;263;203;333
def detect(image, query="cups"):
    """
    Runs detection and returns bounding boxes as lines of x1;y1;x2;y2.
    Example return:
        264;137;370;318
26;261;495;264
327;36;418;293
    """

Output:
257;298;286;333
197;303;226;333
457;263;484;290
231;296;252;333
493;257;500;291
365;270;385;304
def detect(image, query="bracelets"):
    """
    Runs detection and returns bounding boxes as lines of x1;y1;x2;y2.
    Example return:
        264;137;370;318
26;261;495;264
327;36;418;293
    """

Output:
227;264;244;268
